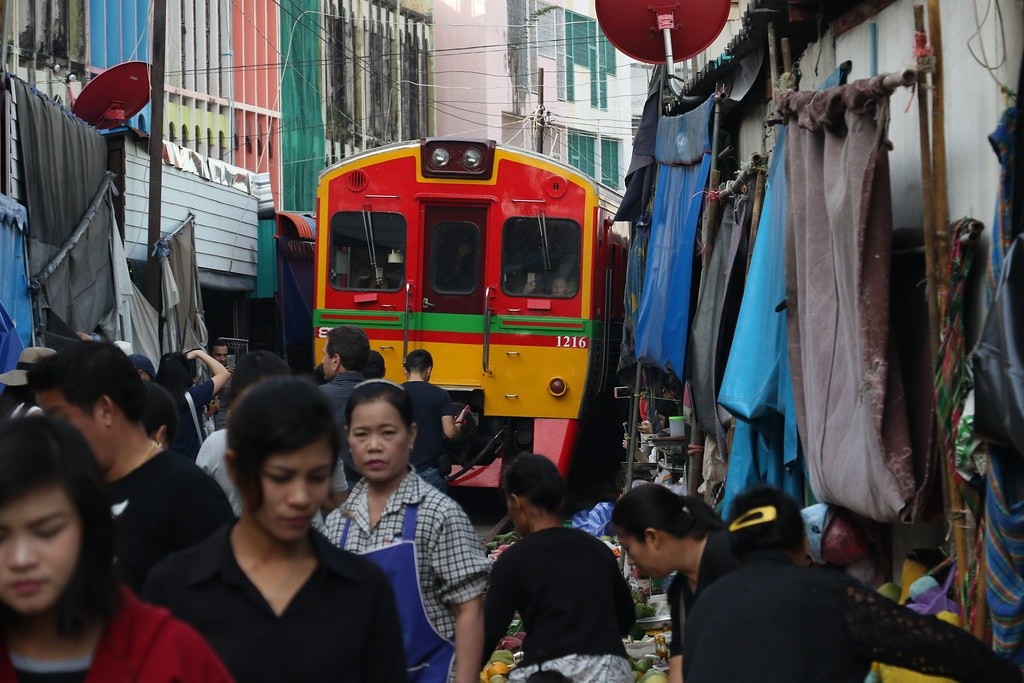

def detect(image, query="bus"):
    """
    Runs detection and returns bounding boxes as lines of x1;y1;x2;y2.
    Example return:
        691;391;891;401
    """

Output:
308;135;634;488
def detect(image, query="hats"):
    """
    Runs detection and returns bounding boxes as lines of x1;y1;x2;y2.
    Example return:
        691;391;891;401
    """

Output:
0;347;58;388
128;352;158;379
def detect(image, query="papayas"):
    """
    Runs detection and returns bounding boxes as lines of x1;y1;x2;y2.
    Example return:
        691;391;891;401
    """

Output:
488;662;508;679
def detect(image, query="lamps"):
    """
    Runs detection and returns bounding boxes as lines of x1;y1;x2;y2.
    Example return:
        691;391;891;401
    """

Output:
44;58;76;86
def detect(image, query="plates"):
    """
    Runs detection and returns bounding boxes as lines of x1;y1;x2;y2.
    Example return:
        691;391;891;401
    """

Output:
635;615;672;629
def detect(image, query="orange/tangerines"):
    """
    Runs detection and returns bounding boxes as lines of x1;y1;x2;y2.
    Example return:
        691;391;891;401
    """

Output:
635;660;647;673
488;675;508;683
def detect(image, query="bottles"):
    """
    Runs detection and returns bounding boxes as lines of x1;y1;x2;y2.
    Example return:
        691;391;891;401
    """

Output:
659;637;668;661
655;638;661;656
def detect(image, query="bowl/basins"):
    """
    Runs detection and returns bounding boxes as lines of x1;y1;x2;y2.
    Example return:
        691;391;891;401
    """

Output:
621;462;658;478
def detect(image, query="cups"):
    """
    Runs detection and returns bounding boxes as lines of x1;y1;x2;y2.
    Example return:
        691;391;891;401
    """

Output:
669;416;685;437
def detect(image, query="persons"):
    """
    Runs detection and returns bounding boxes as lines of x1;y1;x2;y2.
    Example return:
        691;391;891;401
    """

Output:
603;483;740;683
1;340;236;683
521;272;575;296
622;389;686;465
195;348;307;517
682;485;1024;683
138;374;408;683
317;378;494;683
400;348;467;492
318;325;386;474
479;452;638;683
